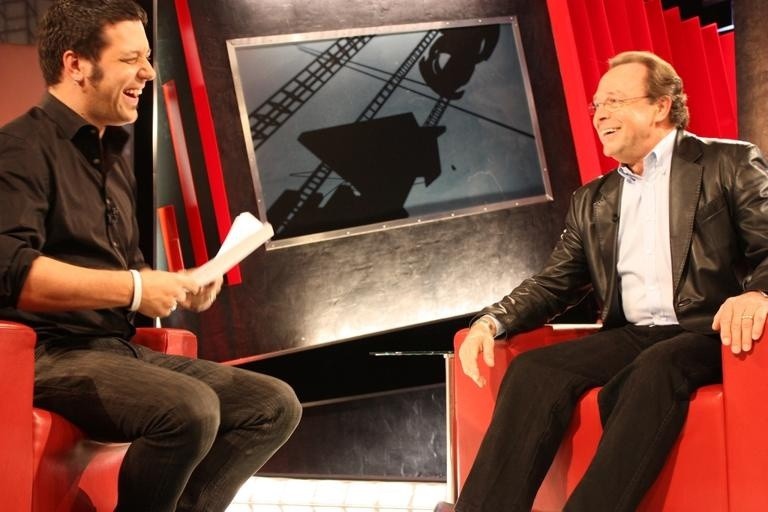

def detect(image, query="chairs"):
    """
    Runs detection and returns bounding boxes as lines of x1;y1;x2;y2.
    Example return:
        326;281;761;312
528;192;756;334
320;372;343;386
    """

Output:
0;319;198;512
452;314;768;512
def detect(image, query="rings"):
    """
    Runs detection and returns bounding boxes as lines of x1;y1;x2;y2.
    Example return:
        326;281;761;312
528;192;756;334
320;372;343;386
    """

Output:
741;314;754;320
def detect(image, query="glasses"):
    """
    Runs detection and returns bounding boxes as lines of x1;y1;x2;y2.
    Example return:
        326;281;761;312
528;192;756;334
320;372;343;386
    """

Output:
586;92;657;113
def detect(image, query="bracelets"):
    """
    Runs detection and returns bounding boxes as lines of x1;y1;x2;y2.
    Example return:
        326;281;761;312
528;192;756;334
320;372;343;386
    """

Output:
128;268;143;314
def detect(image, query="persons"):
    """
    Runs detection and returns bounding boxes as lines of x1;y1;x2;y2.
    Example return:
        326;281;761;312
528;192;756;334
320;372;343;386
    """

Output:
432;47;768;512
0;0;307;511
